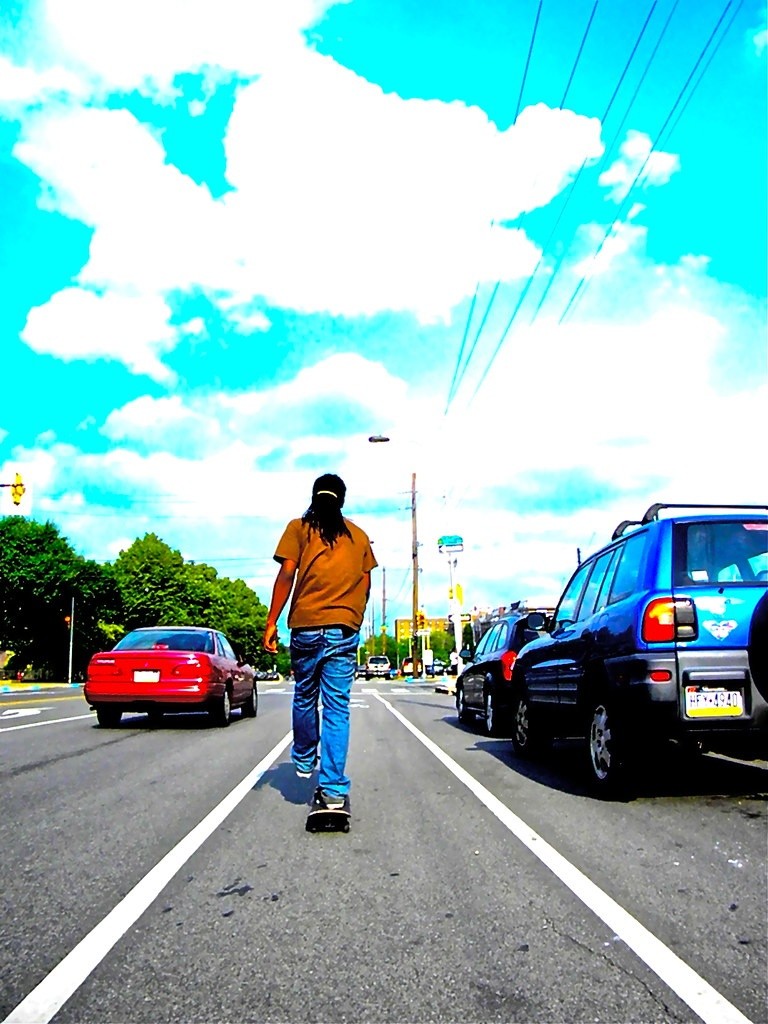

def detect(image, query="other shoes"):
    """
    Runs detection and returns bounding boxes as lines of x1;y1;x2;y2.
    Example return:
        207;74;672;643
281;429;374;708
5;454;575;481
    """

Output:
296;759;318;779
319;788;344;809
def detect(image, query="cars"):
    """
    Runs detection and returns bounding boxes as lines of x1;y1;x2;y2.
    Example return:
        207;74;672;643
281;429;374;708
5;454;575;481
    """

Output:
253;671;279;680
427;663;444;675
357;666;366;677
83;626;257;726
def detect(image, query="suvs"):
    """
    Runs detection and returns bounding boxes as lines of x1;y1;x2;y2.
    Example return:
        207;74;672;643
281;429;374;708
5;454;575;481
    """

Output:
511;502;768;800
366;655;391;681
456;607;561;735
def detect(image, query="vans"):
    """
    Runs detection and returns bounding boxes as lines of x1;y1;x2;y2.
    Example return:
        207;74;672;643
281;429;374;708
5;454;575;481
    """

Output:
402;657;422;676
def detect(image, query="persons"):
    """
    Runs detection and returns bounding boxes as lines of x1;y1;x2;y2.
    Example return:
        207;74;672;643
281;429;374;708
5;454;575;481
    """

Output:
16;660;84;682
261;473;378;809
450;649;458;675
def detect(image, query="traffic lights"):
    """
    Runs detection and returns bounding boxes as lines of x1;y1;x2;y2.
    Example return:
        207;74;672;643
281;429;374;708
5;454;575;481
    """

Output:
418;612;426;629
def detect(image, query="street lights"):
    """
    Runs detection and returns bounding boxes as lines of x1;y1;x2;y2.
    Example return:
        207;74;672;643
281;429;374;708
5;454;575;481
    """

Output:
368;434;418;678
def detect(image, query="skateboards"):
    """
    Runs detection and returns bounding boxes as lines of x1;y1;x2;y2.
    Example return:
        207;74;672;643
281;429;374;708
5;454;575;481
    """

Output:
307;785;352;833
